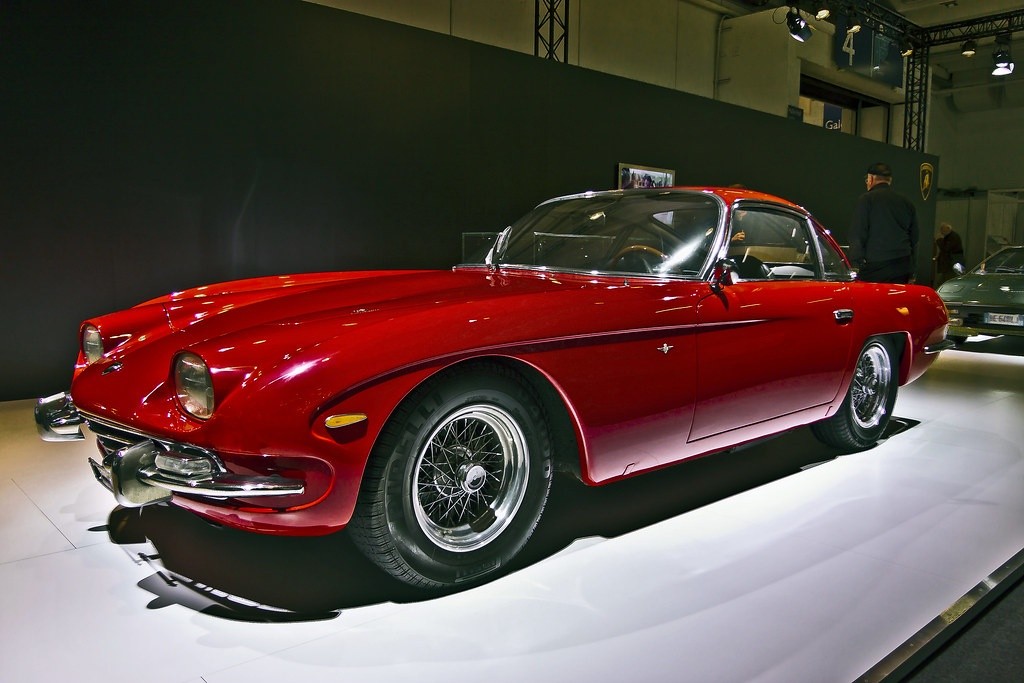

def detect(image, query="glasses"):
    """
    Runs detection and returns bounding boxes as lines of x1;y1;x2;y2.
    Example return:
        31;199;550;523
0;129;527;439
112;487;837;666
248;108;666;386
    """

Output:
865;178;869;183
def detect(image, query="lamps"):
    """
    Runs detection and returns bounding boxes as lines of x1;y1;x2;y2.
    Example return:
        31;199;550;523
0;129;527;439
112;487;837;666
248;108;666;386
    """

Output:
785;6;812;42
897;37;914;57
961;41;976;56
991;36;1015;75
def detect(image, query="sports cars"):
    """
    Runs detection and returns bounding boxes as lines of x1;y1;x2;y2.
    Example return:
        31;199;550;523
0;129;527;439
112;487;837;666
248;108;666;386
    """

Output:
35;185;955;591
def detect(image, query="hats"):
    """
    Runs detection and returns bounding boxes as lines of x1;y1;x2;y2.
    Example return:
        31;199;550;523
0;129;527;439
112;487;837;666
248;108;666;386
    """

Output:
864;162;891;175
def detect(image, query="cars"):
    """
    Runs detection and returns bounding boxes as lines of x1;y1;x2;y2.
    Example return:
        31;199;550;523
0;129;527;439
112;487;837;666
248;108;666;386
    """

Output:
938;244;1024;343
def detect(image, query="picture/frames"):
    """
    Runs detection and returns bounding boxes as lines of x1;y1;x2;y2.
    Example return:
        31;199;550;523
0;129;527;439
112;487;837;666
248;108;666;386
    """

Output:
618;163;675;230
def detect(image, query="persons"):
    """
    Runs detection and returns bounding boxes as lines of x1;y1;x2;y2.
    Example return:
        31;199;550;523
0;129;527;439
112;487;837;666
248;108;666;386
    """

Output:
933;222;964;281
851;163;920;284
621;167;654;189
